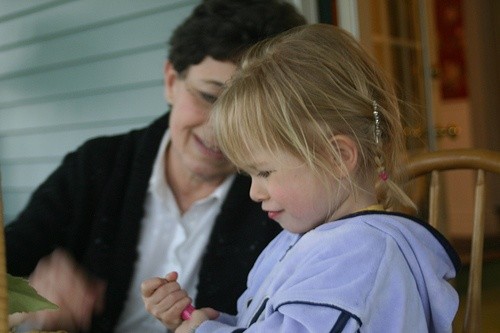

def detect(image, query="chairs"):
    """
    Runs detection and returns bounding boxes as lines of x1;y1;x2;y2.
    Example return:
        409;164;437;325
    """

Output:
373;147;499;333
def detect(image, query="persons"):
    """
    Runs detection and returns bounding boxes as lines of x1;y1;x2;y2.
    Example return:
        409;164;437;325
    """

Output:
140;21;463;333
2;0;311;332
